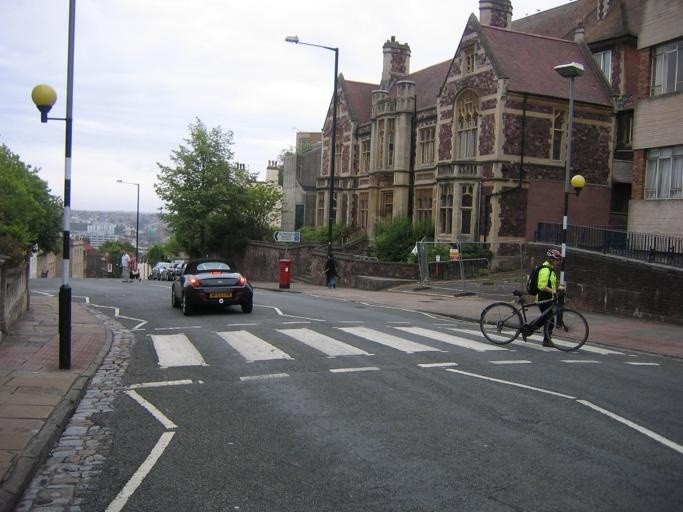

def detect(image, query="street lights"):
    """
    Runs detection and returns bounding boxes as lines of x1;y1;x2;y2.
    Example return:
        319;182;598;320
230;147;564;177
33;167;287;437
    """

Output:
283;34;339;259
30;0;79;369
552;58;584;331
118;179;138;271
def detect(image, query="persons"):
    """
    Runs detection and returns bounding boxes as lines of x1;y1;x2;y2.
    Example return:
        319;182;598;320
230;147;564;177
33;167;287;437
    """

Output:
119;249;129;282
127;252;141;282
520;249;563;347
324;254;337;289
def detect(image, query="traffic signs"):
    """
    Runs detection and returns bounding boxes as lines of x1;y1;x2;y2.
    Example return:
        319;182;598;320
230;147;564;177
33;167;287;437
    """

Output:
272;231;299;243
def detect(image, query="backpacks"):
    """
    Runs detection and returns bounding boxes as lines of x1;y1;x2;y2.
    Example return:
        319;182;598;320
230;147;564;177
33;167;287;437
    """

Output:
527;264;552;296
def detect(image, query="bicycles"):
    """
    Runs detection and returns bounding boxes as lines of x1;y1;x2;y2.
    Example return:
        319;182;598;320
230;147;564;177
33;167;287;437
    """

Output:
475;288;589;352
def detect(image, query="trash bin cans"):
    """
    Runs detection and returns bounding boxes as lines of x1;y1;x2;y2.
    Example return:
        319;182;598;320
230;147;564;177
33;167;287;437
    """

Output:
278;259;291;289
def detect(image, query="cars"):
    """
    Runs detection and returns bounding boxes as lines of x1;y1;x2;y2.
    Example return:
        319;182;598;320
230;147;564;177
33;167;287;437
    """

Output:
152;262;183;281
169;259;254;315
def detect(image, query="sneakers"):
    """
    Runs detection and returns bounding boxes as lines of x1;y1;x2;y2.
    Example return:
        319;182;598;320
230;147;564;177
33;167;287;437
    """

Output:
542;339;554;347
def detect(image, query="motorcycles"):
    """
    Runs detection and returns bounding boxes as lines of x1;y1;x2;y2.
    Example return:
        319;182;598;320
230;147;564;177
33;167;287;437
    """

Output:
147;271;152;279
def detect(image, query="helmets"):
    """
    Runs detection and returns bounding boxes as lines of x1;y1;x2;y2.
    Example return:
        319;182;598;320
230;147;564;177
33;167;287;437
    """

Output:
546;249;561;259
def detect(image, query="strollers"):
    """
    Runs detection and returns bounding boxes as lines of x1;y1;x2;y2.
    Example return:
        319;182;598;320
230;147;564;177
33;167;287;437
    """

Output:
129;264;142;285
